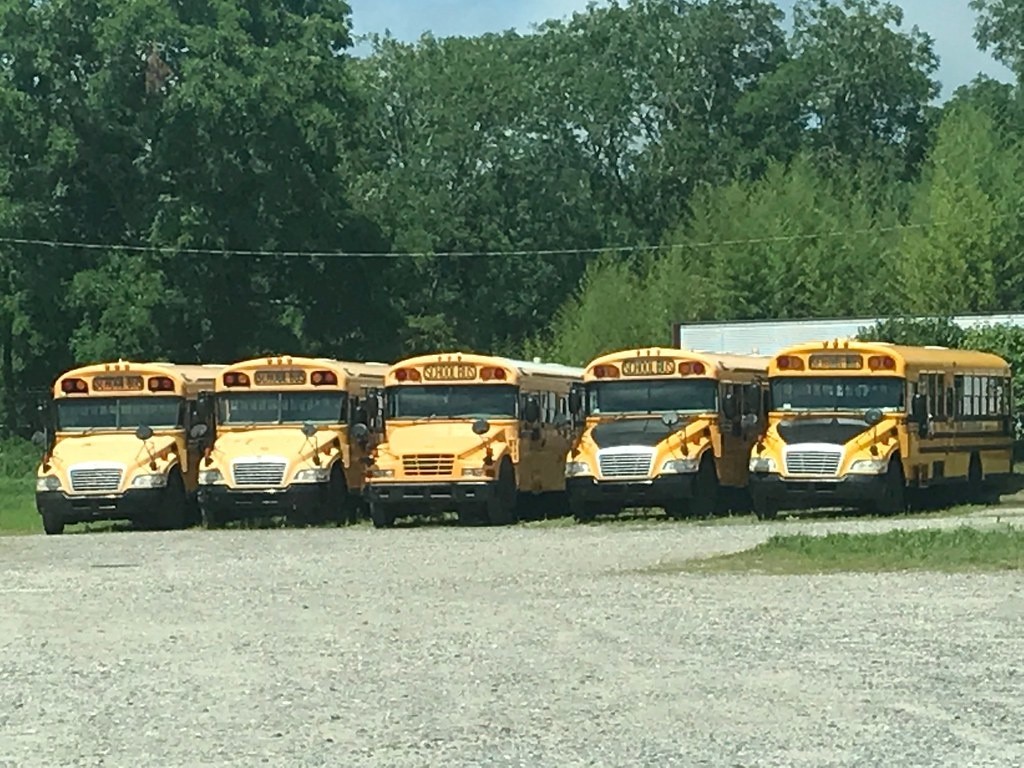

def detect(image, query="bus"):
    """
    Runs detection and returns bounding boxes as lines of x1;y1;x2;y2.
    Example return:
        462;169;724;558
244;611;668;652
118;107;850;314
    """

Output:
747;337;1017;523
353;349;600;529
32;359;240;533
189;353;391;531
564;347;829;526
